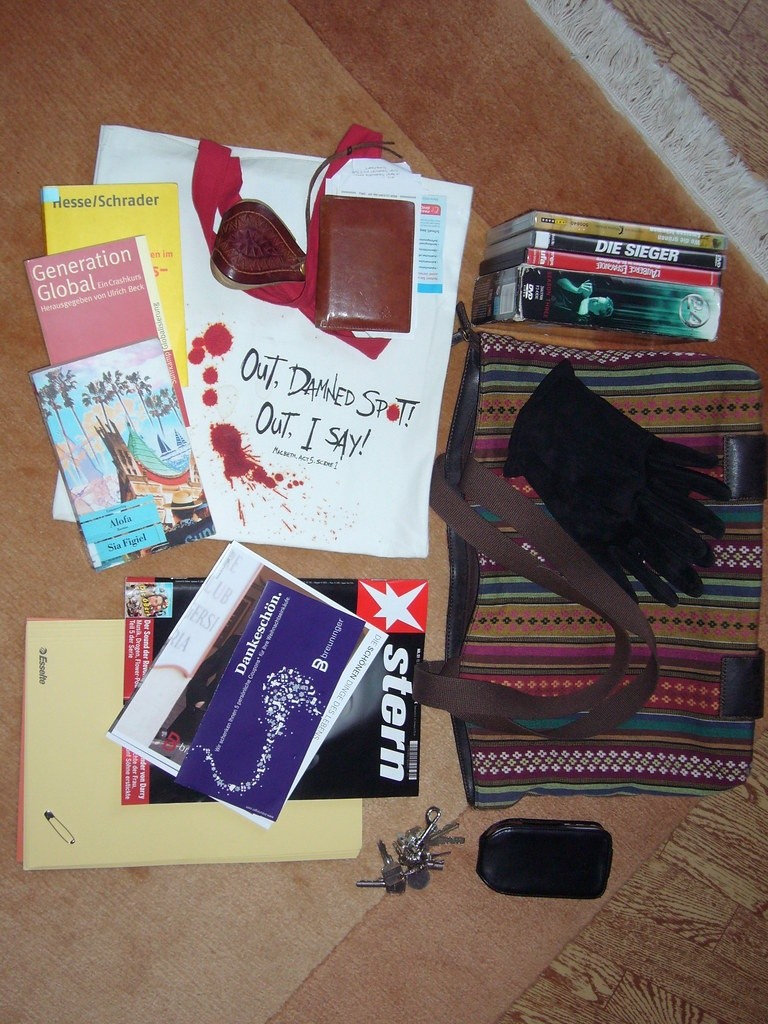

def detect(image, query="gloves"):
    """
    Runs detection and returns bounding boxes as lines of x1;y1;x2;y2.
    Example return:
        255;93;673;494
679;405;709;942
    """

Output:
502;359;732;607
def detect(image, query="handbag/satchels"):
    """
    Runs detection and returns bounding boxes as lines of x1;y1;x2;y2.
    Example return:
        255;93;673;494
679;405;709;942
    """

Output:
412;298;768;806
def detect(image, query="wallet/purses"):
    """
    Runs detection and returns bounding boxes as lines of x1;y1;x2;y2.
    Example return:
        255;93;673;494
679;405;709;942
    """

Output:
318;194;414;333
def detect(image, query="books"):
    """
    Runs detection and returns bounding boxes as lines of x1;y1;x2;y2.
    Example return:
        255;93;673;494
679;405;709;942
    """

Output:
483;208;732;343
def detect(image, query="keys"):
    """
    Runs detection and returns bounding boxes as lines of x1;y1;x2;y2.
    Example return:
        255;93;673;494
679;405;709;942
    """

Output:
356;806;465;893
